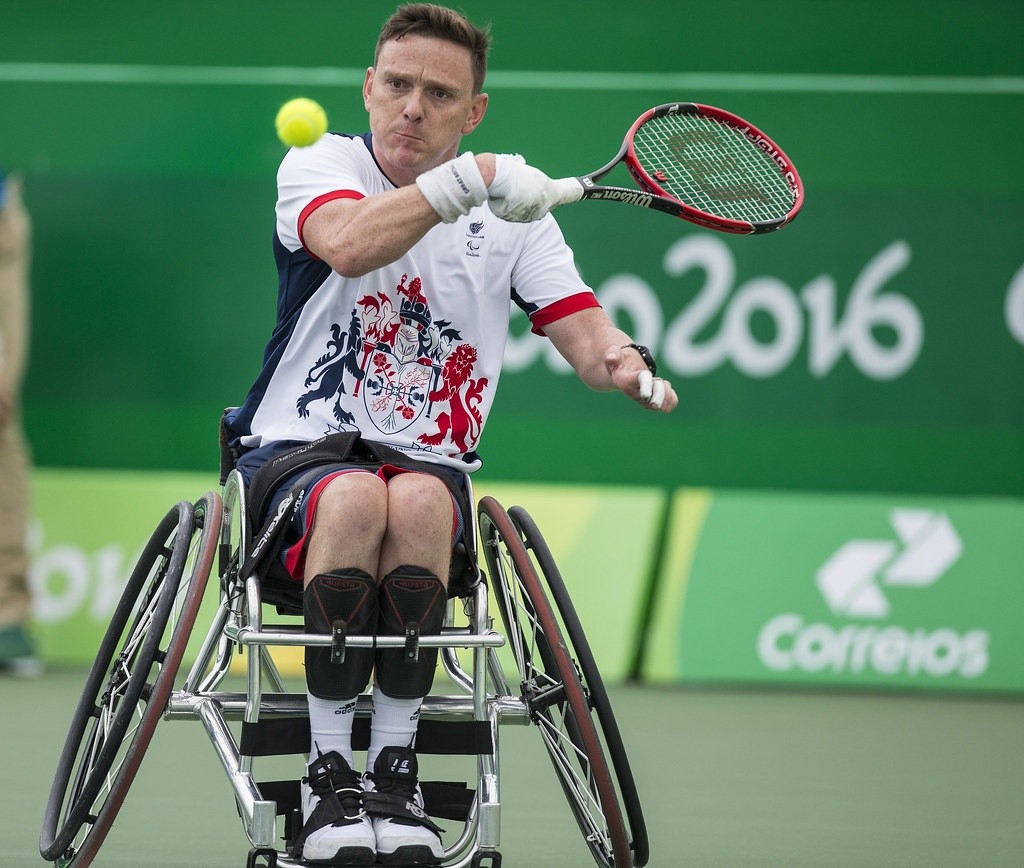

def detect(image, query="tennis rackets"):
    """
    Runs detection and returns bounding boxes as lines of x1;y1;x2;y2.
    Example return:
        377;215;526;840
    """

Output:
486;98;807;237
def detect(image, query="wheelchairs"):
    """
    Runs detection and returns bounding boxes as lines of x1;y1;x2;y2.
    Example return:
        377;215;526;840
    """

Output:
39;403;652;868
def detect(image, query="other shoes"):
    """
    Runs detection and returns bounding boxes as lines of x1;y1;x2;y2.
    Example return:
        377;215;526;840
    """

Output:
0;628;41;674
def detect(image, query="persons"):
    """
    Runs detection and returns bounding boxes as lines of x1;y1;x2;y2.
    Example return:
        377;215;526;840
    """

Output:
0;169;44;687
228;4;679;866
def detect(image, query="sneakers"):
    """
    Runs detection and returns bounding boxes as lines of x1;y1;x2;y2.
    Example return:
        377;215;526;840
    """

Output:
299;740;376;868
362;744;446;868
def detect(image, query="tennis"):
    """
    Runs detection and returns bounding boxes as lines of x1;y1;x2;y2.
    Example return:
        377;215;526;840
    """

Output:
274;95;330;148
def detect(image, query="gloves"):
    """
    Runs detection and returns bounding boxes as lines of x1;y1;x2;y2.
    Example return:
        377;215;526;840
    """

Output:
483;152;564;223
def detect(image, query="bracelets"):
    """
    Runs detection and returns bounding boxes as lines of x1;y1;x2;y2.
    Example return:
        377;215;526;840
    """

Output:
620;342;657;378
